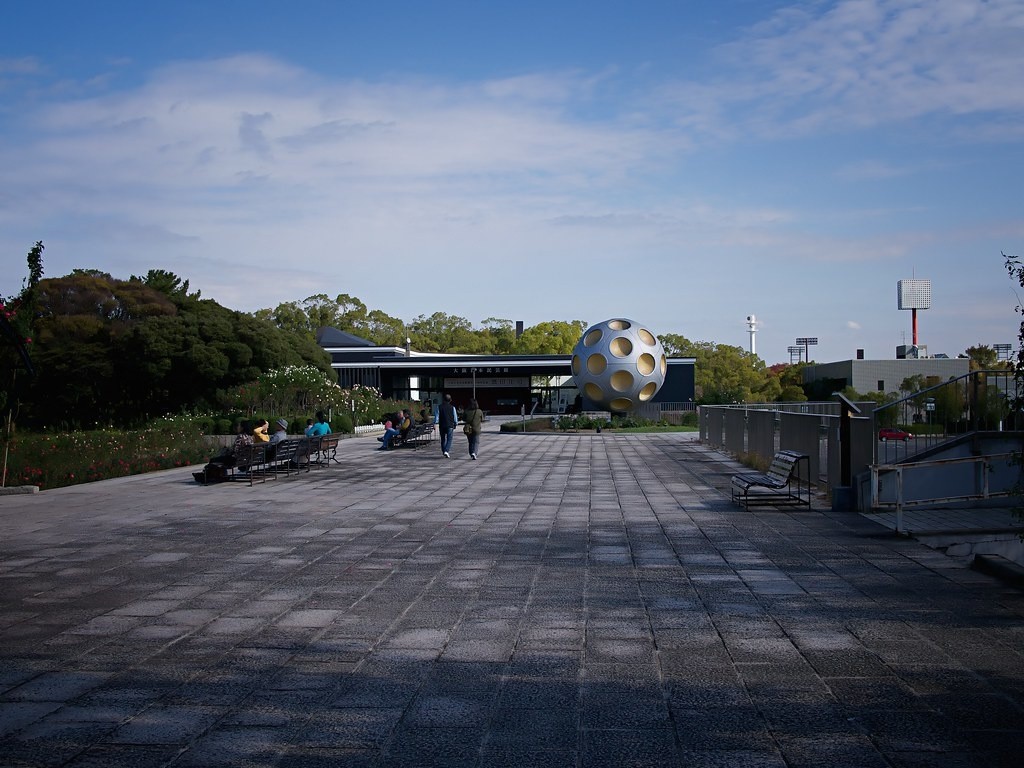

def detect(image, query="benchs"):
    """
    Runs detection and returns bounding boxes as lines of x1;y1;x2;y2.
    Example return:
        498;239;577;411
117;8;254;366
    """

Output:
246;435;320;477
405;423;435;446
306;431;343;467
205;441;278;487
730;449;811;509
387;425;425;451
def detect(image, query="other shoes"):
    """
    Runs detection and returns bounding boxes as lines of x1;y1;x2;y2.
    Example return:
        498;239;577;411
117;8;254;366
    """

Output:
444;451;450;458
192;472;204;483
238;466;249;471
377;438;384;442
378;446;386;450
471;453;478;460
284;460;296;466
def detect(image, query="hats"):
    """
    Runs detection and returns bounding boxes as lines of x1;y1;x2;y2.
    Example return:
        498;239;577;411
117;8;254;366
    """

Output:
276;418;288;430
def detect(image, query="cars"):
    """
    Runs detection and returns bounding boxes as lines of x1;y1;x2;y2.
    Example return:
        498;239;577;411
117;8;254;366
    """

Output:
880;427;913;442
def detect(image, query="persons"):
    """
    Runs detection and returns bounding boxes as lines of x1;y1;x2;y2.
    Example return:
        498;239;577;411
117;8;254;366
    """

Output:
415;410;431;426
304;419;314;436
377;409;413;450
192;420;255;479
269;419;288;458
462;398;483;459
284;411;332;467
397;410;405;426
433;394;458;458
239;419;270;473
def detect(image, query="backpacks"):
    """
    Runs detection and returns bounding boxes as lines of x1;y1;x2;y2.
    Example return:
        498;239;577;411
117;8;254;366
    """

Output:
464;423;472;436
